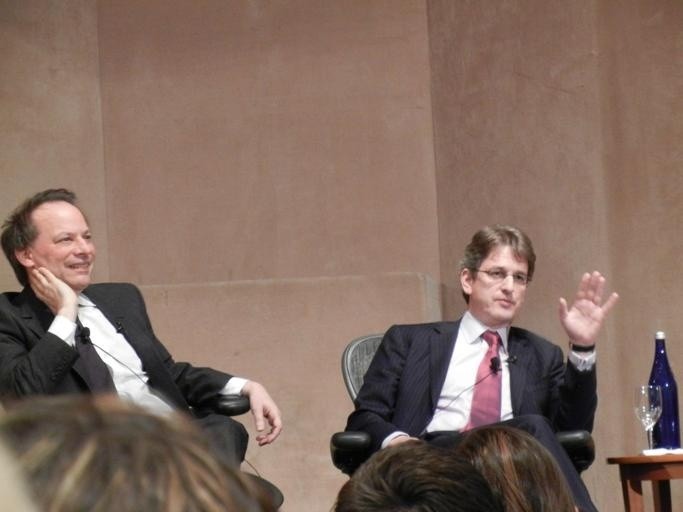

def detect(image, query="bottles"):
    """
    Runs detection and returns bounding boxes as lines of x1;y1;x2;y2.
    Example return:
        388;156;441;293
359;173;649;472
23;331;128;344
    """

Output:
647;332;681;449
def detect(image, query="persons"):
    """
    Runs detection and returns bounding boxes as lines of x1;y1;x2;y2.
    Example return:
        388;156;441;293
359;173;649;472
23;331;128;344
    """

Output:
0;394;274;512
335;425;576;511
343;223;620;512
0;187;282;470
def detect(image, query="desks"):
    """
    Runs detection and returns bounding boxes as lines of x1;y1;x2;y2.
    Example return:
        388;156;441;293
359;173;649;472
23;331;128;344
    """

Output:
605;453;683;511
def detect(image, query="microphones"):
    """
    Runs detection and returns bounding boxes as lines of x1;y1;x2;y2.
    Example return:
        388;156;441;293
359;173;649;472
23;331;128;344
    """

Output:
82;327;90;341
491;357;500;370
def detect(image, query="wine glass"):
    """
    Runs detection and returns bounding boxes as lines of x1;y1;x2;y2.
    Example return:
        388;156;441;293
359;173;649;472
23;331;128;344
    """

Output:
633;385;663;453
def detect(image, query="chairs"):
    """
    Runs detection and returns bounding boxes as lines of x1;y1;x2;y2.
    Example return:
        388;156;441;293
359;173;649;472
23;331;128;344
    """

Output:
328;335;595;478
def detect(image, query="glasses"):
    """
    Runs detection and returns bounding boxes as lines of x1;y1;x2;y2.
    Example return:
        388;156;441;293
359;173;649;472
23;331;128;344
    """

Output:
477;270;531;285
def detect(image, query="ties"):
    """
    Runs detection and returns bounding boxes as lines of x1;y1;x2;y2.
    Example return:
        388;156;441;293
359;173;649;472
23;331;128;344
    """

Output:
461;330;502;433
74;316;118;399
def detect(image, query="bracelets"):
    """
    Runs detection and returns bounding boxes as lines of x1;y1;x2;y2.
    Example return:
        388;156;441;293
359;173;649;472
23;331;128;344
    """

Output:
569;344;595;353
